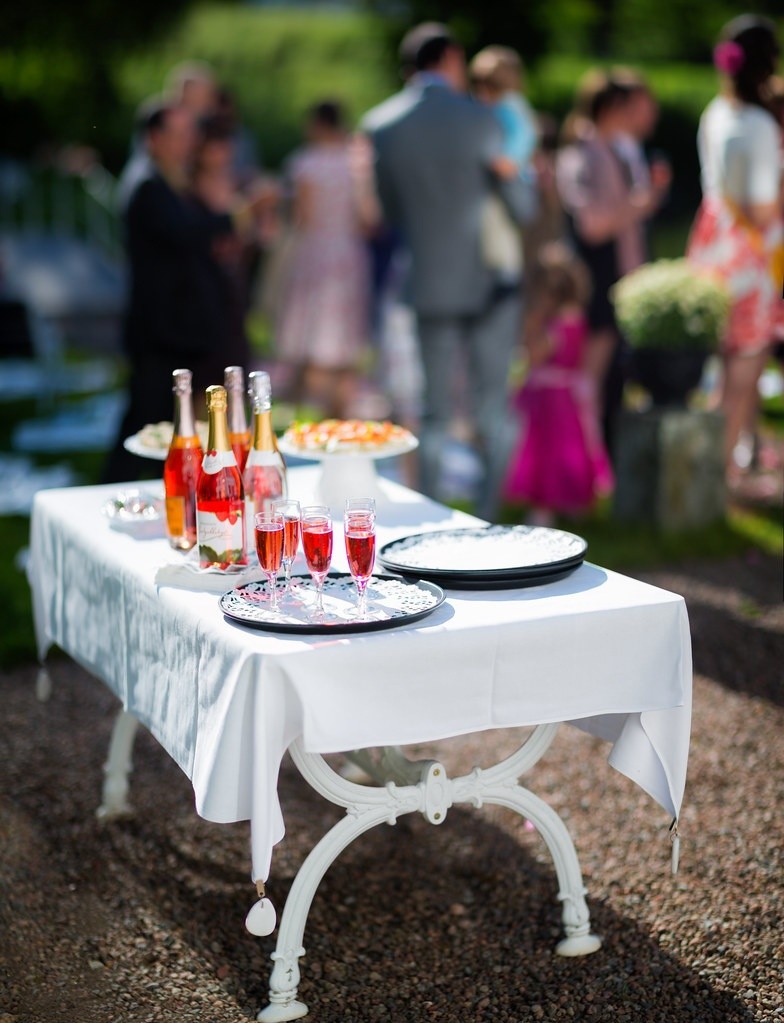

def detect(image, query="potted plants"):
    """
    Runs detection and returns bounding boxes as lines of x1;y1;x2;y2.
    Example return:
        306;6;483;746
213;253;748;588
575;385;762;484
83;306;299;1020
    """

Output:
609;260;735;407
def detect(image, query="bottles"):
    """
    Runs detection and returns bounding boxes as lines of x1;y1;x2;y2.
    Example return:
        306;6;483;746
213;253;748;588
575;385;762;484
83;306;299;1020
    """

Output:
243;372;288;559
224;366;249;476
165;369;203;550
196;385;245;572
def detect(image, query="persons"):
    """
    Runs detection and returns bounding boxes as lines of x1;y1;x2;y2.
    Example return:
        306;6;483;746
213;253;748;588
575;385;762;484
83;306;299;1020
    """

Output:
684;16;784;509
269;98;383;412
356;24;537;522
95;60;279;486
503;241;616;529
552;73;671;449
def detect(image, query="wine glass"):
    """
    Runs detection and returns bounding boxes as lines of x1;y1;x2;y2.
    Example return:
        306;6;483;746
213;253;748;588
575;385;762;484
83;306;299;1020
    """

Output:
255;500;380;623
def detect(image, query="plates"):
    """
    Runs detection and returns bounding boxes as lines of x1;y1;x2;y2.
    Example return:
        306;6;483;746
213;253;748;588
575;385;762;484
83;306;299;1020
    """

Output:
124;430;210;460
277;422;418;462
219;573;446;634
376;526;588;591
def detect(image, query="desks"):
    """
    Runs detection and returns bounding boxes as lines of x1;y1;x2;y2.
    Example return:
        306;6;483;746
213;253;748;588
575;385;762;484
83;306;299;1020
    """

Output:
28;461;692;1023
